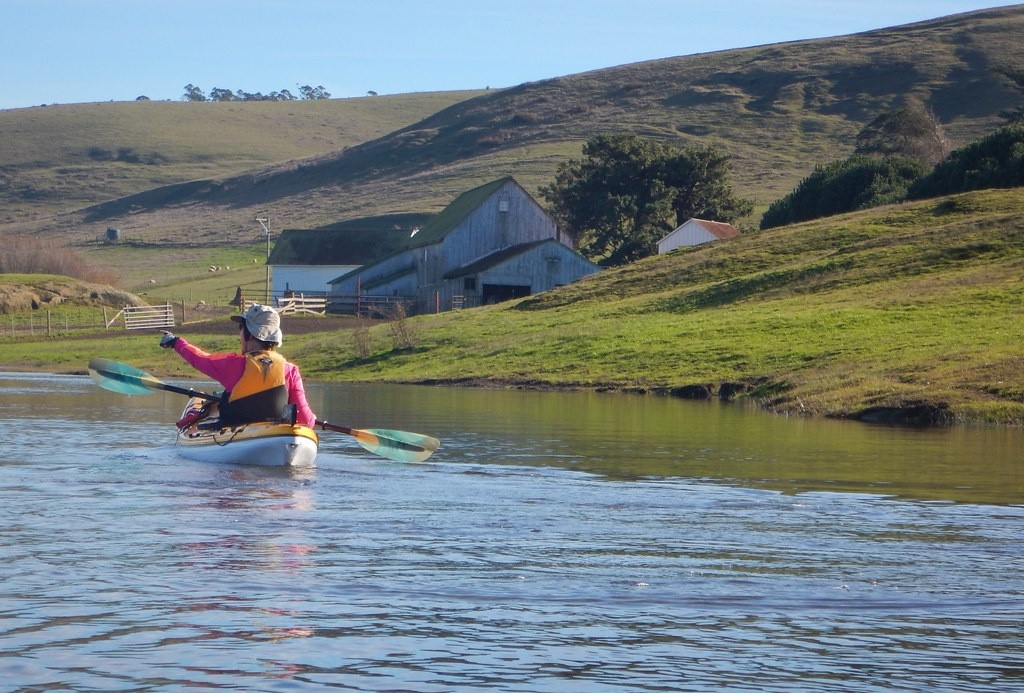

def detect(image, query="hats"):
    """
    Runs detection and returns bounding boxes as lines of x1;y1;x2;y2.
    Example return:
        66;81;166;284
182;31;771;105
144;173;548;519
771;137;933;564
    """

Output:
230;303;282;348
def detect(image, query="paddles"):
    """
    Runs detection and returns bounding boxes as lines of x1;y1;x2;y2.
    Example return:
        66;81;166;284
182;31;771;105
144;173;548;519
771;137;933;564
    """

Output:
87;355;441;463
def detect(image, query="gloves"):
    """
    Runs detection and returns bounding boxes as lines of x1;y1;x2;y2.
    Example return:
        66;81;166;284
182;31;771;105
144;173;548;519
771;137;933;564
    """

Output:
158;329;177;348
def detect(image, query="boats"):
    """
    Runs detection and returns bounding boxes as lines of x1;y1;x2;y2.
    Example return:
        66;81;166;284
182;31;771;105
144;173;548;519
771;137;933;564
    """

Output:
175;396;319;472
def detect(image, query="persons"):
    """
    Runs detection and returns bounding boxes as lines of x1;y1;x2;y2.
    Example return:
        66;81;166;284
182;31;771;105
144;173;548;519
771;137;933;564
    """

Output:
159;304;316;430
